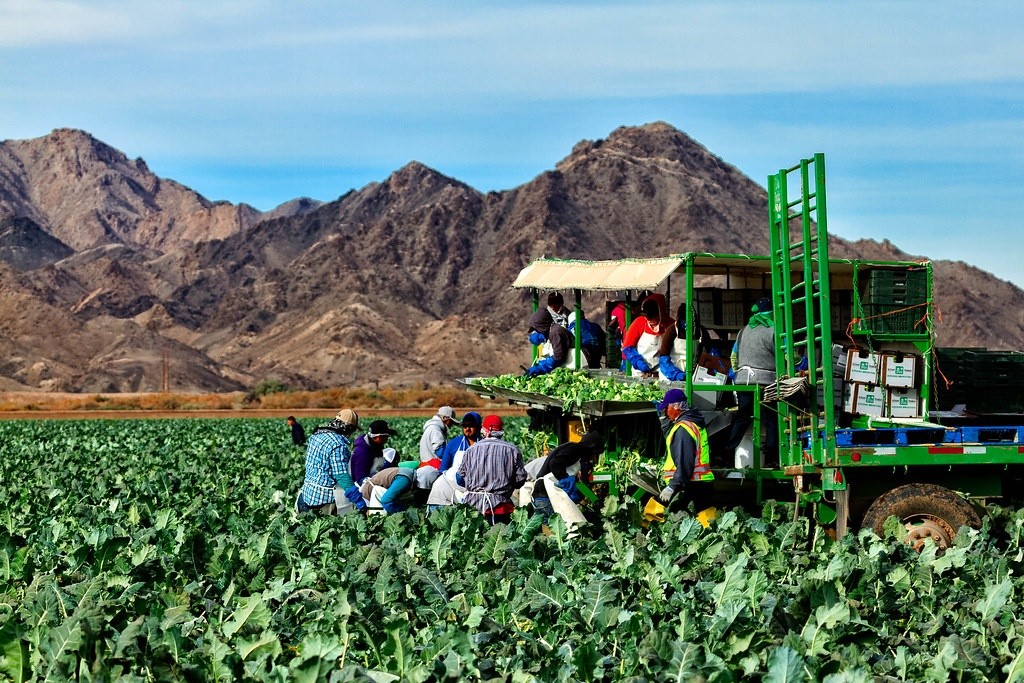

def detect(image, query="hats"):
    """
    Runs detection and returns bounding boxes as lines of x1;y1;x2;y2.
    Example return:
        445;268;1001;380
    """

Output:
528;327;535;333
756;297;773;312
336;409;363;431
460;413;477;428
481;415;503;431
437;406;459;425
382;448;400;463
368;420;397;435
589;431;605;465
548;292;563;303
568;310;584;327
640;300;659;316
657;389;687;410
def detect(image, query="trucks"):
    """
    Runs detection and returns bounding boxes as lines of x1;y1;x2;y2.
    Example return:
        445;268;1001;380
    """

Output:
459;154;1023;562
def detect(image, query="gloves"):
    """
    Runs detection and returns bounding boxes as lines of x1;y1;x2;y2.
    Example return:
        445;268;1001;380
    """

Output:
659;486;674;502
623;346;650;372
523;356;555;376
659;356;686;381
360;506;367;518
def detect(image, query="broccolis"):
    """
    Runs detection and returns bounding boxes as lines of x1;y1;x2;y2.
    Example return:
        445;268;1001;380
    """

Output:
468;367;673;492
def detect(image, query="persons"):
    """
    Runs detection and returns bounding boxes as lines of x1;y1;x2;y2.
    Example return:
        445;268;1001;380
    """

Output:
288;416;306;446
717;299;808;468
524;292;724;380
653;389;715;515
297;406;606;523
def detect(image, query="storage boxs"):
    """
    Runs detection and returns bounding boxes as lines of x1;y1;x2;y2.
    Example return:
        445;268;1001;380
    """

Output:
880;354;916;390
844;349;881;386
843;381;886;417
693;266;927;334
691;352;731;411
884;387;919;418
605;300;637;369
820;343;847;377
816;376;843;409
929;346;1024;415
